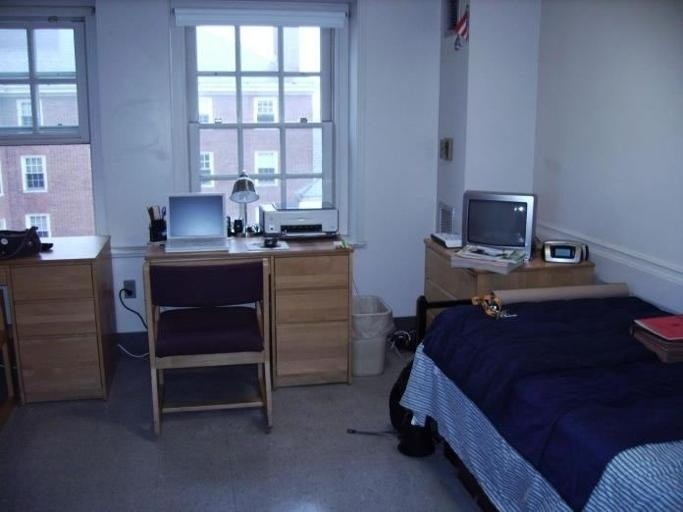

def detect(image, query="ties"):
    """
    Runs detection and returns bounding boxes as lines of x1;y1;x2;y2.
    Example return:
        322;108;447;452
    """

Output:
462;189;538;261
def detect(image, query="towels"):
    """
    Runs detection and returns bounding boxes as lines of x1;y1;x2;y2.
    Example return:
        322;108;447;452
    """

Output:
350;295;393;377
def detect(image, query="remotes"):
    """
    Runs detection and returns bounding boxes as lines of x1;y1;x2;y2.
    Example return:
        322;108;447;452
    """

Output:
264;236;277;247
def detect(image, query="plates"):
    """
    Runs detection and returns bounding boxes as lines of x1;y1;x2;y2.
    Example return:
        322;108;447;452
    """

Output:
421;237;596;338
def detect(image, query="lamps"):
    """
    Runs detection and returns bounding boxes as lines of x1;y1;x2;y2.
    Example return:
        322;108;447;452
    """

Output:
141;259;276;435
0;290;14;400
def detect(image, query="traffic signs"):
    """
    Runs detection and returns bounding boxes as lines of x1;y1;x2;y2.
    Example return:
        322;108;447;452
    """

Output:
121;280;134;299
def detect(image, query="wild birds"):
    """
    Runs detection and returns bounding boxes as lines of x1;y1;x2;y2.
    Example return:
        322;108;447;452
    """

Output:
164;192;228;253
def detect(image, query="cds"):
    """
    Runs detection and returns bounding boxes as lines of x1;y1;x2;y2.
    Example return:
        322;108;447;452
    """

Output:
488;302;518;320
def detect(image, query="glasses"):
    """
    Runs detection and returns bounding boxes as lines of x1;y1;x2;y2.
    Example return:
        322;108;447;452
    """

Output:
228;169;259;229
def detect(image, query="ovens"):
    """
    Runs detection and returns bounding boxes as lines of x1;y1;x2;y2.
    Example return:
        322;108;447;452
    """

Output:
413;280;683;512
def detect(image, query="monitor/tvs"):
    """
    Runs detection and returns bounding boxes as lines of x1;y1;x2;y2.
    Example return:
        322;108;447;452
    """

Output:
449;244;528;275
633;315;683;363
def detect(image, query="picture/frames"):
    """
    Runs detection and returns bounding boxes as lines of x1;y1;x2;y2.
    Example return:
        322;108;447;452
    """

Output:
0;236;113;409
145;232;353;392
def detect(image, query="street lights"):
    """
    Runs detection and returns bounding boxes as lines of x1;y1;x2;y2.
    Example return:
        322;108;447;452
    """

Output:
0;226;41;260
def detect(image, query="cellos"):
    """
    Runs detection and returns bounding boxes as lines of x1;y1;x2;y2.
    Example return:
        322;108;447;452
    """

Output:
258;201;339;241
